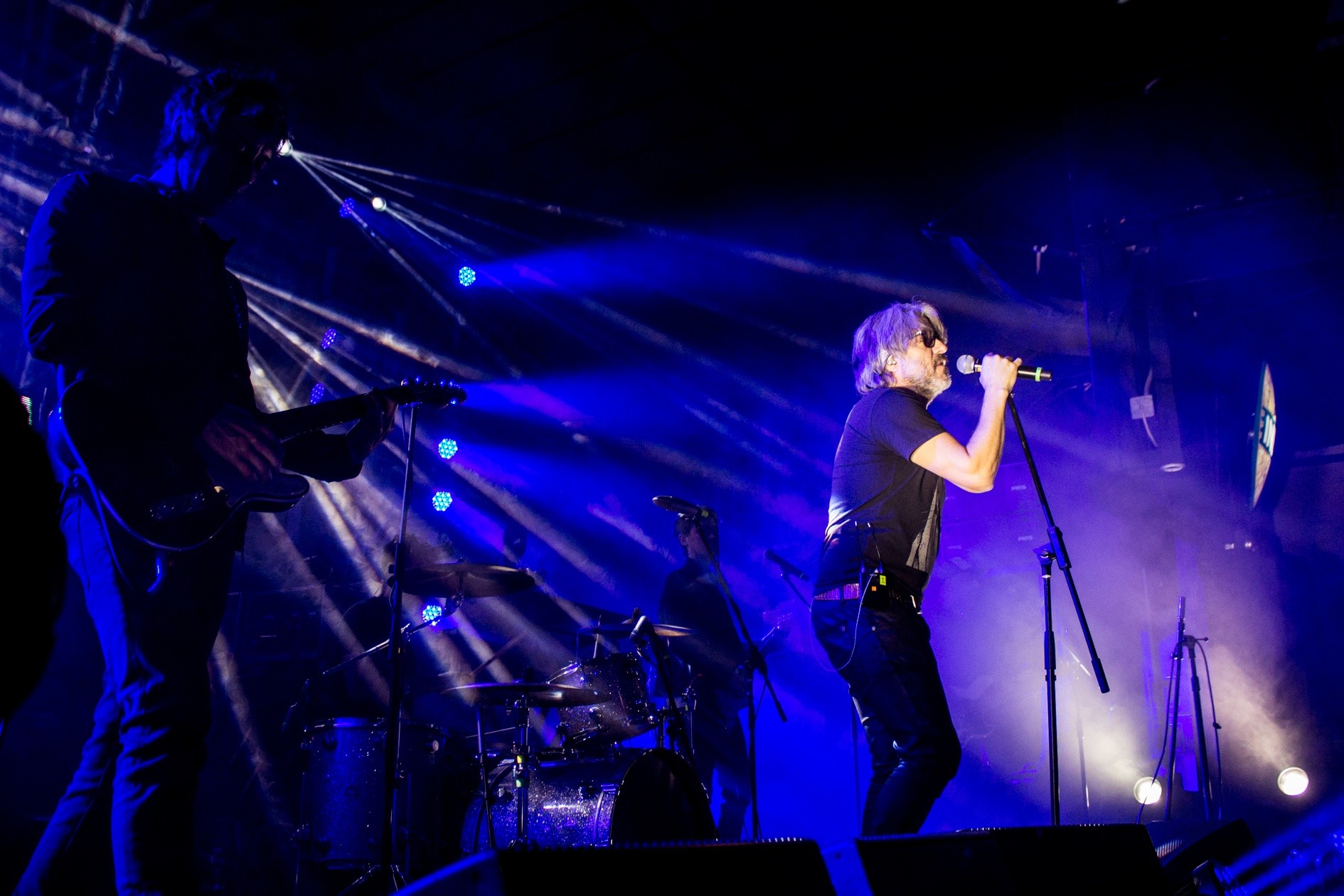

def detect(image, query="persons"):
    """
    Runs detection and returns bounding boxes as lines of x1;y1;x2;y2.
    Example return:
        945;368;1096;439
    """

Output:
658;507;769;842
15;61;397;896
812;303;1022;838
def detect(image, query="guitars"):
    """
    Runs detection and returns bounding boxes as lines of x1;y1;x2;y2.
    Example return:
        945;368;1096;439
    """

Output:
686;642;777;738
60;374;468;553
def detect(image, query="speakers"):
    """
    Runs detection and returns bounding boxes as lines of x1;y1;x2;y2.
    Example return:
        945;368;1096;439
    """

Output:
836;824;1173;896
387;836;836;896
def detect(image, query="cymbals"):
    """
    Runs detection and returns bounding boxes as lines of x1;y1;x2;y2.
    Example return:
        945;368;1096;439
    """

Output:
435;680;611;707
577;621;694;639
386;560;536;600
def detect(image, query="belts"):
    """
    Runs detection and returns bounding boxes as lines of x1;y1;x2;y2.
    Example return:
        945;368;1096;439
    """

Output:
815;583;861;600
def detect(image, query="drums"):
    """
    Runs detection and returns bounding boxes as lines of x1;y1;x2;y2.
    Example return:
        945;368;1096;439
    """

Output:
458;748;720;851
297;714;449;874
549;652;660;748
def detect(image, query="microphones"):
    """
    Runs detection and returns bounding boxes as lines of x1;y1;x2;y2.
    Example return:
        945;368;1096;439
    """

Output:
762;549;809;581
956;354;1052;382
652;496;710;519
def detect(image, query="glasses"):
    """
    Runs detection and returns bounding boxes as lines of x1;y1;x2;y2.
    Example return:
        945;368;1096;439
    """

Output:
899;328;945;348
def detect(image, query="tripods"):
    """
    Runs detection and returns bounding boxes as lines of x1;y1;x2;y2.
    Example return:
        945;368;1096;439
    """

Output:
335;408;419;896
